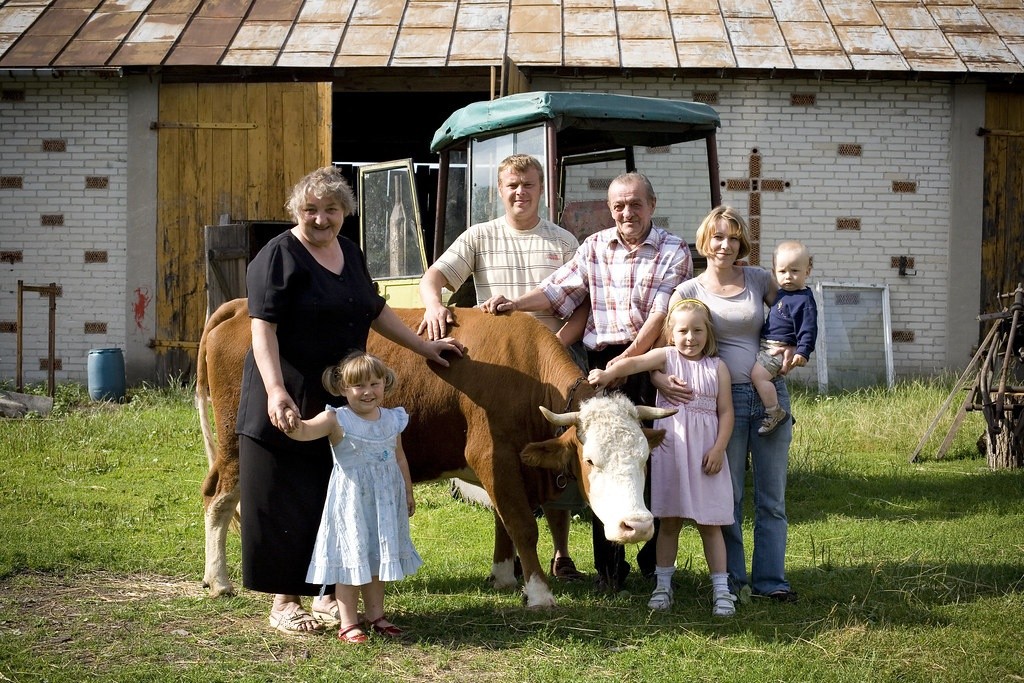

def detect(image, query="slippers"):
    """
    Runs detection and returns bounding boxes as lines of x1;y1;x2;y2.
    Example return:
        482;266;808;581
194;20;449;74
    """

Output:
314;605;365;625
269;612;322;636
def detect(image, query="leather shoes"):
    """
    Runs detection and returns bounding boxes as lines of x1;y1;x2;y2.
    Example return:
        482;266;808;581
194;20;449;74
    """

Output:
365;616;404;637
338;624;370;644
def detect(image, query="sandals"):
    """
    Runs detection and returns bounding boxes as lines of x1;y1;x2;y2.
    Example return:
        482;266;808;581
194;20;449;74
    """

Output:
647;587;675;611
712;590;736;618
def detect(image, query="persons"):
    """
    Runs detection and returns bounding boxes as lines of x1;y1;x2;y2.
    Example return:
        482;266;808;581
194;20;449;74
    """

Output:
479;168;694;600
587;298;737;617
416;154;591;584
284;351;427;645
751;241;819;439
650;205;799;605
233;166;464;638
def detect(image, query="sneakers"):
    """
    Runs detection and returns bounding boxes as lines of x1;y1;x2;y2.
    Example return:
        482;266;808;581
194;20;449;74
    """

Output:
758;406;790;436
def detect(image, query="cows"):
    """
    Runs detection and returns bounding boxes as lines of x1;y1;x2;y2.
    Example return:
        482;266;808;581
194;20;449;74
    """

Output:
197;297;680;612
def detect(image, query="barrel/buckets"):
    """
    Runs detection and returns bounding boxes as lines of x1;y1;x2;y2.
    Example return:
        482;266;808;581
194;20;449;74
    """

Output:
87;347;126;402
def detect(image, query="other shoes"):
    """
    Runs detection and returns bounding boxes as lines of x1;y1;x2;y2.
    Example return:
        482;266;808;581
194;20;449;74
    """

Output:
731;593;742;605
751;589;798;605
600;579;630;595
642;571;679;589
514;557;522;577
551;557;584;584
